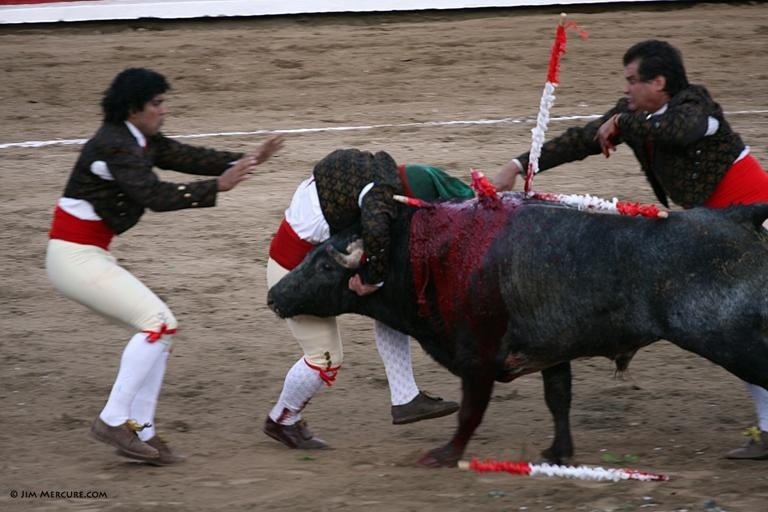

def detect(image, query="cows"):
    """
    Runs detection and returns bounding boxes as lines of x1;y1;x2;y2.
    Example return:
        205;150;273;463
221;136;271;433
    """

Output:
266;200;768;468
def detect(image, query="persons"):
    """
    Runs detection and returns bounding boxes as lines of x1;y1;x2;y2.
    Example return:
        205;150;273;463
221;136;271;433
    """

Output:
45;68;287;467
487;39;768;458
264;147;477;452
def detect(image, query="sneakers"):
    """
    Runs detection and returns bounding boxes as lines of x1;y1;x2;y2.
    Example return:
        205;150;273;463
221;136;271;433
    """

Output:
263;414;333;450
391;389;459;424
725;425;766;460
87;415;186;467
